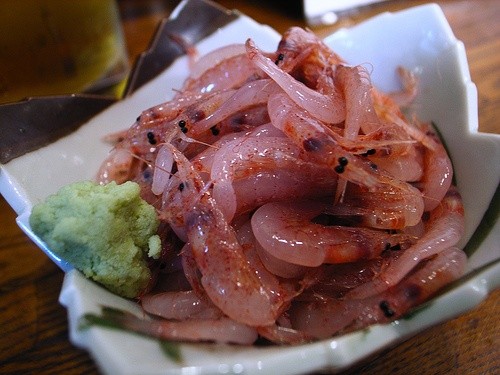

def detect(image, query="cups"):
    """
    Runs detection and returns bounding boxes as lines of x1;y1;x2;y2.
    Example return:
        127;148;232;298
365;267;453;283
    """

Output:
0;1;148;107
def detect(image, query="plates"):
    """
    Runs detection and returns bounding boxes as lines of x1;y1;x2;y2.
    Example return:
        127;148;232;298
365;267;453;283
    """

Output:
0;0;500;375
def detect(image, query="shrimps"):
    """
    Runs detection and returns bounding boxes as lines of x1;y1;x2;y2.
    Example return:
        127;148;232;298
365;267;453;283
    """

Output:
95;26;469;346
